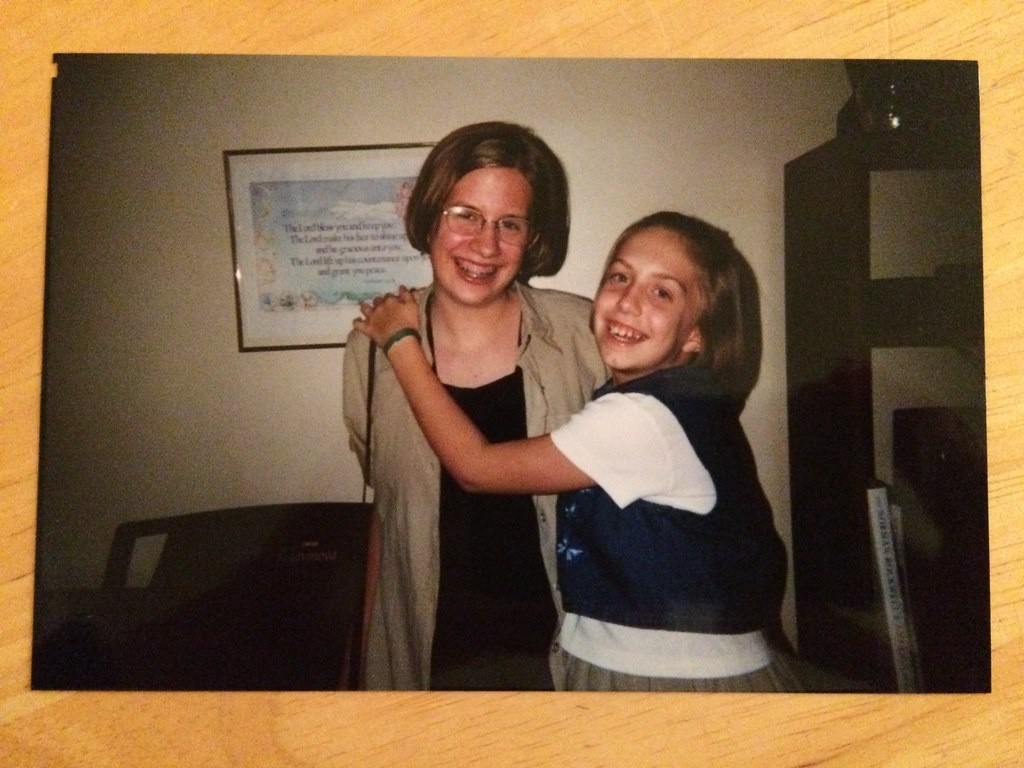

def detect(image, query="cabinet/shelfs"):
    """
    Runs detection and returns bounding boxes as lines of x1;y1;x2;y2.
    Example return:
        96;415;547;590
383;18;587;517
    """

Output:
784;115;991;691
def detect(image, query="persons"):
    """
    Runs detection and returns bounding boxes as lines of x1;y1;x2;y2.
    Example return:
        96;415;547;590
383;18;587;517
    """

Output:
357;211;855;694
342;122;607;691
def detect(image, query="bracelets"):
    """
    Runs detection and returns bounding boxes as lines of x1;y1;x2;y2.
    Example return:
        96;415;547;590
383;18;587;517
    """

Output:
382;328;422;358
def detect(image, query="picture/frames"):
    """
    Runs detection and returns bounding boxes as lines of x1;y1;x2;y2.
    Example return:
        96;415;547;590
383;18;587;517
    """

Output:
223;142;447;352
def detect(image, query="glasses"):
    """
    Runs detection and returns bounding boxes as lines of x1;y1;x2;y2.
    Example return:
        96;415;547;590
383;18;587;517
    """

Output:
440;204;542;248
